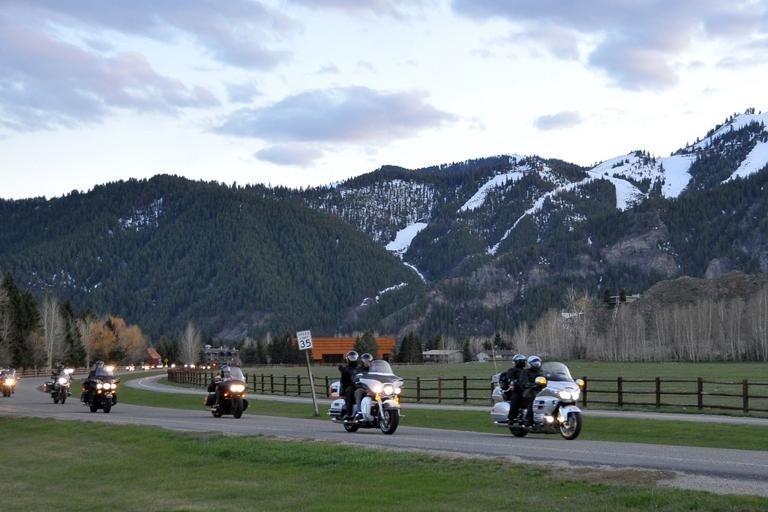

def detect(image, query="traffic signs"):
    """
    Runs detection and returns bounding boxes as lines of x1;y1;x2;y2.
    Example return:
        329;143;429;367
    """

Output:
296;330;312;350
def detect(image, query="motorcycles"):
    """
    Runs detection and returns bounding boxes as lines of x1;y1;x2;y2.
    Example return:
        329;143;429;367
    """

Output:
327;360;407;434
204;367;248;418
82;364;120;413
489;361;585;440
0;368;16;397
43;371;72;404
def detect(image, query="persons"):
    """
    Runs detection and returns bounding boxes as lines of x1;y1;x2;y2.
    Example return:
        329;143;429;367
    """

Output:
211;365;232;407
86;359;107;405
352;351;374;421
51;363;68;393
338;349;365;420
499;354;526;422
519;352;545;425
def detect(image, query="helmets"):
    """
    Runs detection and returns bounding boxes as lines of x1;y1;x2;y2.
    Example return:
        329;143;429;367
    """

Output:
527;355;543;369
221;364;231;372
96;360;104;368
57;364;65;370
346;351;360;361
360;353;373;363
512;354;527;368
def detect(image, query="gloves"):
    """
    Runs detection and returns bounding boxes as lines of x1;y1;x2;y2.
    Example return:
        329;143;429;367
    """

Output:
529;385;541;392
